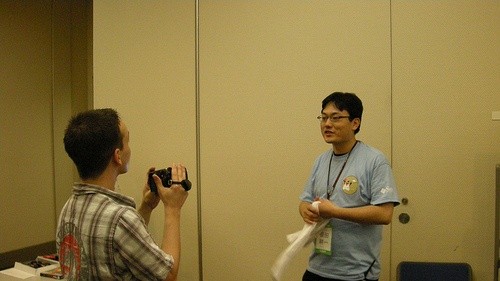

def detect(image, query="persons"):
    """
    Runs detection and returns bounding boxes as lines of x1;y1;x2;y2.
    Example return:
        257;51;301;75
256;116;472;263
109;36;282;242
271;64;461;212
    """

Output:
54;107;189;281
297;91;401;281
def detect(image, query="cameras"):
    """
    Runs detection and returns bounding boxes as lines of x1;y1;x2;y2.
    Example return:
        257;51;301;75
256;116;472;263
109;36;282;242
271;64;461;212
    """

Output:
148;168;188;192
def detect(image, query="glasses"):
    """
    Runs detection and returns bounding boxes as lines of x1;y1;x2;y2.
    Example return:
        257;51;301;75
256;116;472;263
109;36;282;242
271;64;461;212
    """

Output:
317;114;352;121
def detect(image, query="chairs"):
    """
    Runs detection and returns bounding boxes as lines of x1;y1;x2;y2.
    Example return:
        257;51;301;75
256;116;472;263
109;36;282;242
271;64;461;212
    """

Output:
395;262;472;281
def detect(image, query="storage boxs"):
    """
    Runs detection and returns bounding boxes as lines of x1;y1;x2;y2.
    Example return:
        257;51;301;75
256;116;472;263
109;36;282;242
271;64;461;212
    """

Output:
0;256;60;281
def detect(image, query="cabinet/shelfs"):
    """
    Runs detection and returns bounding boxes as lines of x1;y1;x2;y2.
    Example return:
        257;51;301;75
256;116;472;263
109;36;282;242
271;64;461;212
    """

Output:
89;0;500;281
